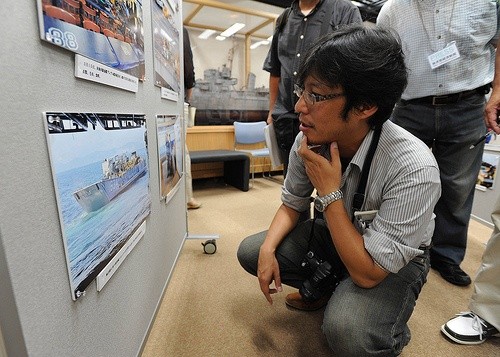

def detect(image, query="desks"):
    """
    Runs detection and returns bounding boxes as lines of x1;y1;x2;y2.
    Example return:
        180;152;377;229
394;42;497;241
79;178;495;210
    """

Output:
189;150;250;192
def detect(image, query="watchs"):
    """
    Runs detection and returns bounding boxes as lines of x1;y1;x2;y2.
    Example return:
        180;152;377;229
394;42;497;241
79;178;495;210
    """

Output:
314;189;343;212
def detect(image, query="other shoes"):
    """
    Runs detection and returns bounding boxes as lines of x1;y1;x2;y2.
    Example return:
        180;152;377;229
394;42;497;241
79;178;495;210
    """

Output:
285;292;325;311
186;197;202;209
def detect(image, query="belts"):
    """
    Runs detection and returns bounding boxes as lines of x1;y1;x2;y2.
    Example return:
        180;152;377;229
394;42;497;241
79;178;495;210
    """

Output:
400;83;492;106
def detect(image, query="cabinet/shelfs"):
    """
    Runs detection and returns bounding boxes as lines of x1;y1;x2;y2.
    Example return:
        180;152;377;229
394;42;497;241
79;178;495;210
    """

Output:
469;144;500;229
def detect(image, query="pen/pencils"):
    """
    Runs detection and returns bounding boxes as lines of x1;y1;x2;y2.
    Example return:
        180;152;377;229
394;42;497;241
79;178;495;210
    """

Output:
469;129;494;150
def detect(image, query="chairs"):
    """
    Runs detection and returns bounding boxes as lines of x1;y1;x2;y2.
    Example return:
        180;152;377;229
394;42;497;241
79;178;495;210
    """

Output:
234;121;270;185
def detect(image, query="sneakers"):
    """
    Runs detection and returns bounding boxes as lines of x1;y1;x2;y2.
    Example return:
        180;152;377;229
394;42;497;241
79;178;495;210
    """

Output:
441;309;499;345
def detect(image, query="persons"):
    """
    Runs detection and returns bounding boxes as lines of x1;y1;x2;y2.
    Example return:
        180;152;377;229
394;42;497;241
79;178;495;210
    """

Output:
376;0;500;287
441;34;500;345
236;23;442;357
183;26;201;210
263;0;365;220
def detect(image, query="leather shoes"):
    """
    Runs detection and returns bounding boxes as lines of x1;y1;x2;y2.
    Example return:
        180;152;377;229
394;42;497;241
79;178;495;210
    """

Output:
430;256;472;286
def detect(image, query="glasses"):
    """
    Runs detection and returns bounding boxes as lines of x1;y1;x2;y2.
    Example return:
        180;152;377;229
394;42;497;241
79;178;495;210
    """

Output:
292;82;345;105
